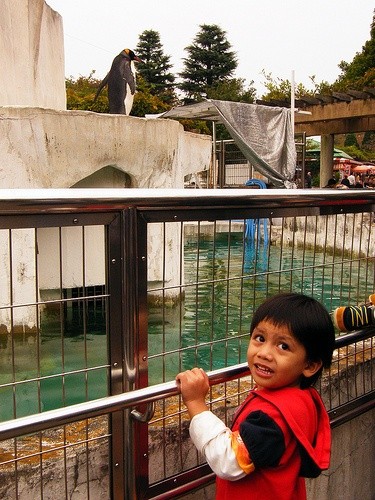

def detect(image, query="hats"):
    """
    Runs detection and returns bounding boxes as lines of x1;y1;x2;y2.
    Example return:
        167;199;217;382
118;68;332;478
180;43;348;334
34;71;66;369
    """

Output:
328;178;338;185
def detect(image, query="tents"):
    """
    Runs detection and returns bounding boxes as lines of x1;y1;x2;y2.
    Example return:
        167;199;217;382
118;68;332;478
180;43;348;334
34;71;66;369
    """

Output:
306;138;375;176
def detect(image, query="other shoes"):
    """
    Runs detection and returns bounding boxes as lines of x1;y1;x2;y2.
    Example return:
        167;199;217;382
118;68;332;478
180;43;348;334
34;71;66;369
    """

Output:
332;304;375;332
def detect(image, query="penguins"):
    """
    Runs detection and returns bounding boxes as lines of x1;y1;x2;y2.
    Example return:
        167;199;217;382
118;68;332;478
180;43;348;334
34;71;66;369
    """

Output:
91;48;144;115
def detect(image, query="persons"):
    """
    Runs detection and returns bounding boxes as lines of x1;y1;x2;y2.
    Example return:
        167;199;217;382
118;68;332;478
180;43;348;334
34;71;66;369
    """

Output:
334;293;375;331
175;292;336;500
307;171;375;190
